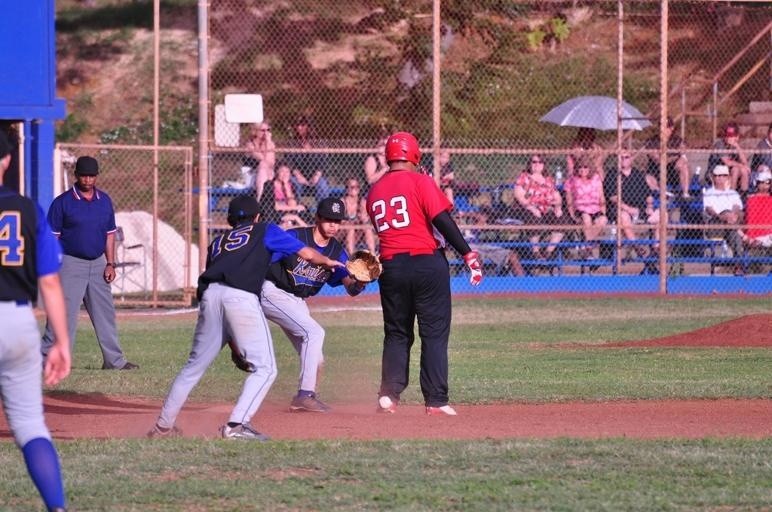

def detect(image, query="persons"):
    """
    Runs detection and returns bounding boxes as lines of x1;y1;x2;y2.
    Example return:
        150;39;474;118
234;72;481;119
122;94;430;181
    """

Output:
0;123;69;512
238;112;771;274
146;193;346;440
232;197;367;413
39;155;140;372
366;132;482;420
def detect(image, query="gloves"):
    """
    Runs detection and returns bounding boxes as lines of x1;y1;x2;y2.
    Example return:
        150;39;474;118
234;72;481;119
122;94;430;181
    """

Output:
463;249;482;286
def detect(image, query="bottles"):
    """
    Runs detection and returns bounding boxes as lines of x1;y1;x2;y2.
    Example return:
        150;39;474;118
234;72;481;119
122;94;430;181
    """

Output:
610;221;617;242
554;166;563;189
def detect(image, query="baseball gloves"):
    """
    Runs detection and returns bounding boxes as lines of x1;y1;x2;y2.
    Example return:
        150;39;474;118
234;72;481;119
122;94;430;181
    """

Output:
345;250;383;284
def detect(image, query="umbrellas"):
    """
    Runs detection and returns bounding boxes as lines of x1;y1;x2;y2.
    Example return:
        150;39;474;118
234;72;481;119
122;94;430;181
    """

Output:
541;92;651;132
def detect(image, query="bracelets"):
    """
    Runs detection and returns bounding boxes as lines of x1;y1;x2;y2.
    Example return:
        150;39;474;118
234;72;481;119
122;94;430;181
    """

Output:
106;262;117;268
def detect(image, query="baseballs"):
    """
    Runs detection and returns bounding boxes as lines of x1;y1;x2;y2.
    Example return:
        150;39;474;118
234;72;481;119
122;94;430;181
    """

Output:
380;395;391;410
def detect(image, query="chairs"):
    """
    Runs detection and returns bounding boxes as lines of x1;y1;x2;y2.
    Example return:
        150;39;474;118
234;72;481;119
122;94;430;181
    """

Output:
115;227;148;299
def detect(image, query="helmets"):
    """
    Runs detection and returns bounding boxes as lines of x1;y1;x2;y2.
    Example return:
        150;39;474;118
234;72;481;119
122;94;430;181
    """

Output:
385;132;421;167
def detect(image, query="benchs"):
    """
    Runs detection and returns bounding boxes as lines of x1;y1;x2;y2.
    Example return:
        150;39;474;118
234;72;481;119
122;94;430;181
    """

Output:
180;183;772;275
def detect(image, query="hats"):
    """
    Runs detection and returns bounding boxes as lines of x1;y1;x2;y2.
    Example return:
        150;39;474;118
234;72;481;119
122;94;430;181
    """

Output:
228;193;264;217
714;123;767;182
76;156;98;176
317;198;349;221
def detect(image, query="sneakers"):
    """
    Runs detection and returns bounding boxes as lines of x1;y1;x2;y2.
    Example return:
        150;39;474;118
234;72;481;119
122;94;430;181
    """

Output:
426;404;457;417
121;363;139;371
289;393;334;413
146;425;183;441
218;421;272;441
375;394;398;414
